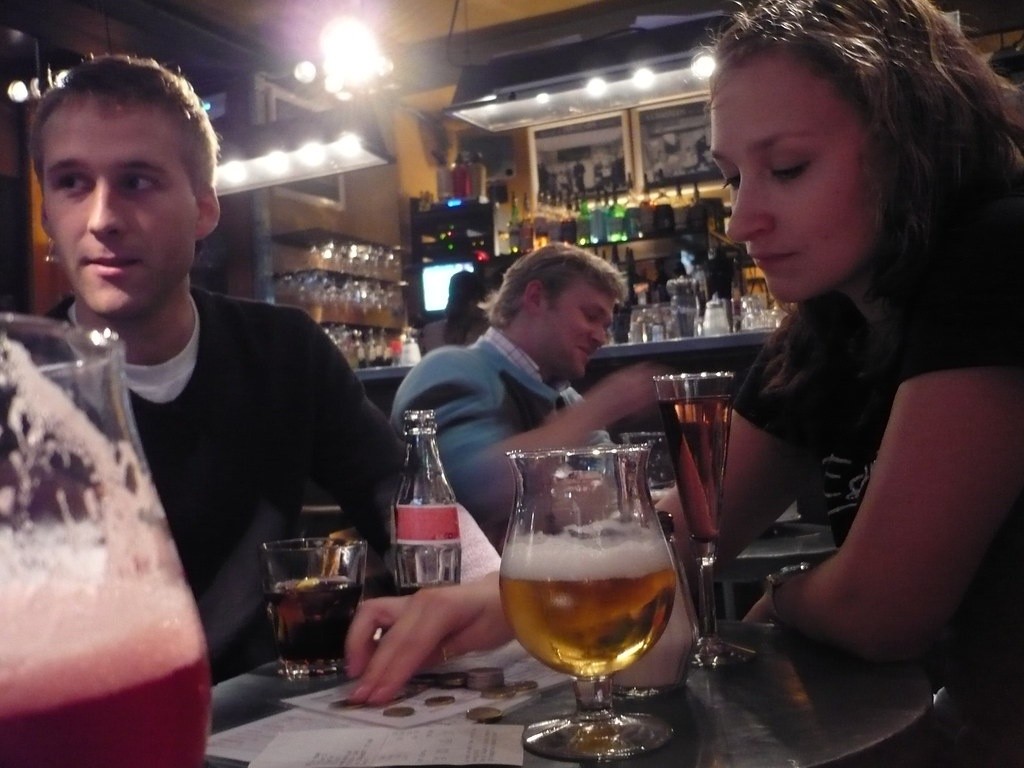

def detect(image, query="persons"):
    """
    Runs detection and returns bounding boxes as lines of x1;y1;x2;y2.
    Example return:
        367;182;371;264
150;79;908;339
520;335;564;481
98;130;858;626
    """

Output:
343;0;1024;768
0;53;505;732
385;243;679;546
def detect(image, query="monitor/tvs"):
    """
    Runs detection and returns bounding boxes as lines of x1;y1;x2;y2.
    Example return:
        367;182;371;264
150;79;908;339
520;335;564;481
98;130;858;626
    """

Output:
421;259;480;315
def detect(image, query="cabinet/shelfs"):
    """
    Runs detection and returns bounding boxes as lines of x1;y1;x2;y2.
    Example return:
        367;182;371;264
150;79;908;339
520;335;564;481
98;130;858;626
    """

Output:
272;227;407;330
526;93;771;313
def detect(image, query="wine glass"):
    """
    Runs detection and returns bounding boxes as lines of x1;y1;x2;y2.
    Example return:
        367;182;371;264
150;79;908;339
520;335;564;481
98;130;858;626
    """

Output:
651;371;753;669
499;444;682;762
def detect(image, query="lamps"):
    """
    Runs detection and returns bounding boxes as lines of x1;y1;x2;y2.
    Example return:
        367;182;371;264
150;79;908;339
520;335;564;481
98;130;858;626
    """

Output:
210;95;398;197
442;16;741;133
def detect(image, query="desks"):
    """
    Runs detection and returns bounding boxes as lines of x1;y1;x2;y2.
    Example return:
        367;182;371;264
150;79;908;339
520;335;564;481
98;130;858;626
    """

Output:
199;619;932;768
711;522;839;619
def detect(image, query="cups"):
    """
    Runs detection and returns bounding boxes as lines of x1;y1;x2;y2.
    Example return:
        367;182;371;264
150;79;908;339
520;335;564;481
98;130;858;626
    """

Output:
0;309;212;768
262;537;368;680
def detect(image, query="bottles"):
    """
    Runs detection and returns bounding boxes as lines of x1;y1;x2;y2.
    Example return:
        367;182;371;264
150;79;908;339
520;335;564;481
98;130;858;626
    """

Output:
355;329;394;368
390;409;463;593
509;182;704;253
432;150;486;196
612;512;699;710
